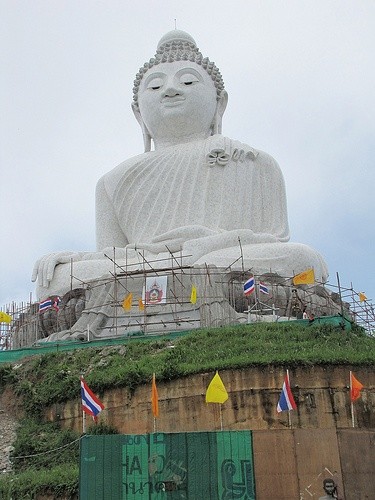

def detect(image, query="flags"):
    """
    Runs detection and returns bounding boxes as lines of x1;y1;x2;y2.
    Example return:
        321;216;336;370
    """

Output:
52;296;62;316
122;291;132;312
259;280;269;295
276;372;298;414
139;296;145;312
152;374;160;417
0;312;13;325
244;276;255;295
293;269;315;285
360;293;367;304
350;373;364;401
81;375;105;426
205;373;229;404
39;297;52;315
190;287;196;305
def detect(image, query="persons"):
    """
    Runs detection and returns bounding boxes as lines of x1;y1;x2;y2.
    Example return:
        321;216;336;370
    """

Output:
30;30;330;300
